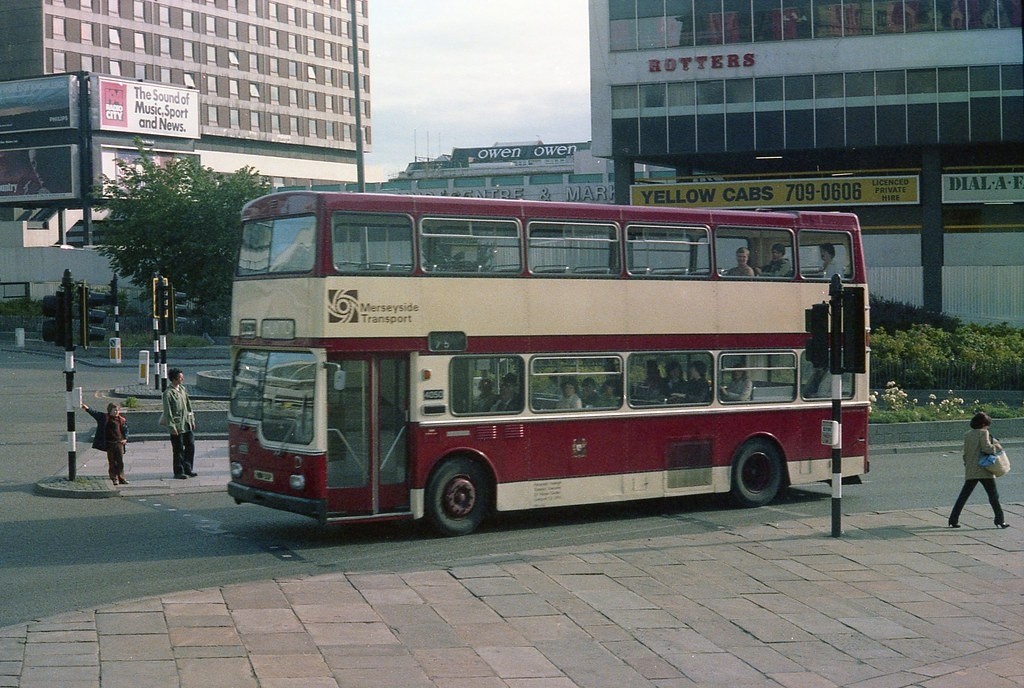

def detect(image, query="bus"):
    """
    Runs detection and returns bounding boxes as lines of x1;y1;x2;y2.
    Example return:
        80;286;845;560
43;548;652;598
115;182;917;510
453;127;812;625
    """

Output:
227;187;873;537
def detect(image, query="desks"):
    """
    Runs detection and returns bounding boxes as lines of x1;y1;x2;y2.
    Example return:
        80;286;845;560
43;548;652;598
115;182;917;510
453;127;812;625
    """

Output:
571;265;610;273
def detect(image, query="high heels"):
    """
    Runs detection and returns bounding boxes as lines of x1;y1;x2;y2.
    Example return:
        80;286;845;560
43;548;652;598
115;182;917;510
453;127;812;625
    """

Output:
948;520;961;528
994;520;1010;529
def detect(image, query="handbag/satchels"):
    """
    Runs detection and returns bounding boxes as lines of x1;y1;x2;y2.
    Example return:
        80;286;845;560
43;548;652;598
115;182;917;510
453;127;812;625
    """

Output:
159;413;167;426
983;452;1010;477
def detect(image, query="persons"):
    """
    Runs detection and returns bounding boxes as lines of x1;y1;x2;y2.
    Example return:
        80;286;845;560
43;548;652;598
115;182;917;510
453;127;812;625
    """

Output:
473;369;522;413
542;368;621;411
717;364;754;404
945;410;1012;529
725;247;753;277
754;242;791;278
638;355;711;405
816;244;846;281
161;368;198;480
80;403;130;484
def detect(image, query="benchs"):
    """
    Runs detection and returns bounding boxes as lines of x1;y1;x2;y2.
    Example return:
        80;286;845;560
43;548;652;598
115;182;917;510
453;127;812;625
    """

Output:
533;264;568;272
356;261;412;272
787;265;827;278
722;266;762;276
532;383;731;409
490;263;521;272
612;265;651;274
651;266;688;274
424;261;485;272
691;267;724;275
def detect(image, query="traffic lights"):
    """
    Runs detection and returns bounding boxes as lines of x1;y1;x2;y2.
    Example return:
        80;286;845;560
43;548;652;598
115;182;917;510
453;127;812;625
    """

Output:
804;304;829;369
83;287;107;349
42;291;66;347
170;283;189;334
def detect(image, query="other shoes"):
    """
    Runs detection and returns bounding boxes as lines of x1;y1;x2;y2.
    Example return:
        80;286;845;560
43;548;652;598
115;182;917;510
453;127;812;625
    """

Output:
174;474;188;479
113;480;118;484
187;472;197;477
120;479;129;484
